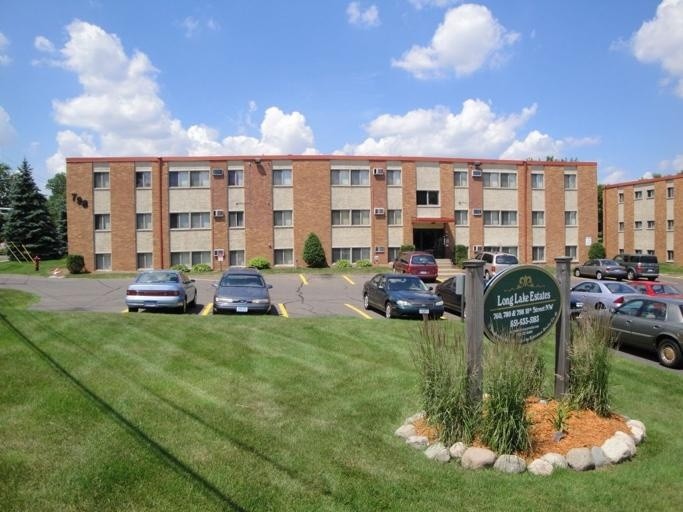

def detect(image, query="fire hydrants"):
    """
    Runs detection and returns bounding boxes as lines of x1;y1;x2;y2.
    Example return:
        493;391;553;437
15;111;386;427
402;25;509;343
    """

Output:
33;255;41;271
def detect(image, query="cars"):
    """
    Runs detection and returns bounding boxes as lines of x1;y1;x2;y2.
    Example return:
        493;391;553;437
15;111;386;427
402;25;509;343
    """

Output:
363;273;444;322
434;276;485;319
125;270;199;315
573;259;627;281
210;270;274;316
568;278;683;371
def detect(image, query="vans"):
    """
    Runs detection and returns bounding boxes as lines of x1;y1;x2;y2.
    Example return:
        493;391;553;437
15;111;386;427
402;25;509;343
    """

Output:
391;249;439;281
610;253;660;281
473;251;519;281
